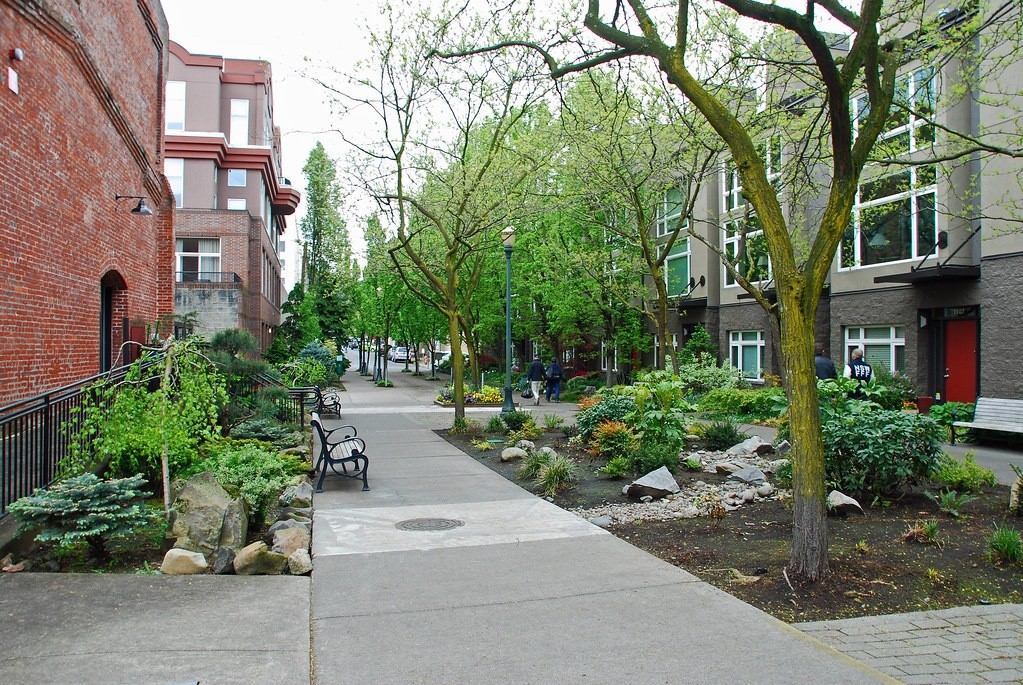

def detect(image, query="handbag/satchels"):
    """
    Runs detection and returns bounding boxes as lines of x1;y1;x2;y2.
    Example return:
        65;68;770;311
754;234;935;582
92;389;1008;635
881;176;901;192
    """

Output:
521;382;534;398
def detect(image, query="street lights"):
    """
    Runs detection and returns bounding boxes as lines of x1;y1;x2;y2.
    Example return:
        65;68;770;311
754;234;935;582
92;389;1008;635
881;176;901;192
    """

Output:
501;224;516;420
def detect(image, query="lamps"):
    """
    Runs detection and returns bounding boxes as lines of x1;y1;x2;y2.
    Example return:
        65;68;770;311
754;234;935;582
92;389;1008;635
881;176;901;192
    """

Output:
757;255;767;266
868;233;890;262
115;194;154;215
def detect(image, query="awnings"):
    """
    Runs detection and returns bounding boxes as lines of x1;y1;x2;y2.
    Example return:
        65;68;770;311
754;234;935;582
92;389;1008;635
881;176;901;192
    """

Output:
737;261;829;299
651;282;707;307
874;225;980;283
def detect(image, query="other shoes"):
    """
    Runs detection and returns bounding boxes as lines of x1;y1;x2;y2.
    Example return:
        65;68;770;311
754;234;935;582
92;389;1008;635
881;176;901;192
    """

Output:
534;397;540;406
555;400;559;403
545;396;550;402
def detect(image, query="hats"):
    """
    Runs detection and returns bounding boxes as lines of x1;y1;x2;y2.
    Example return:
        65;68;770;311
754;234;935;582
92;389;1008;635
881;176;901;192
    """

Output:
534;354;540;358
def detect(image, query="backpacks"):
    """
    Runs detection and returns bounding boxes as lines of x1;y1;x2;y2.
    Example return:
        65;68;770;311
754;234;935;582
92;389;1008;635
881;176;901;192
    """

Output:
548;364;560;383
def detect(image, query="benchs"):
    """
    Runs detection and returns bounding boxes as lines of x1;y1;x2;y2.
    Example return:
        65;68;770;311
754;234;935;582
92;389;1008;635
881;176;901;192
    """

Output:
309;410;370;492
314;385;343;418
947;396;1023;446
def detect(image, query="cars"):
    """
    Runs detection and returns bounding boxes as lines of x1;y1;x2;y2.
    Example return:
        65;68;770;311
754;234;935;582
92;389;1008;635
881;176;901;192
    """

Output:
378;345;392;357
348;337;385;352
387;347;397;360
392;347;411;362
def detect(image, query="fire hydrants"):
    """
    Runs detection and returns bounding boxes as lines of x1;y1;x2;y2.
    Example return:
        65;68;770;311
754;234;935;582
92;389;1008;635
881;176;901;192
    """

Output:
424;356;428;365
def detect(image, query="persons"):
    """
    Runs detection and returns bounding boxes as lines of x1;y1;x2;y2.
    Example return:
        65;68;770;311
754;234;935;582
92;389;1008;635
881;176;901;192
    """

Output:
424;355;428;364
526;354;547;405
351;343;356;350
815;343;838;416
546;358;562;403
464;358;470;366
842;348;875;401
